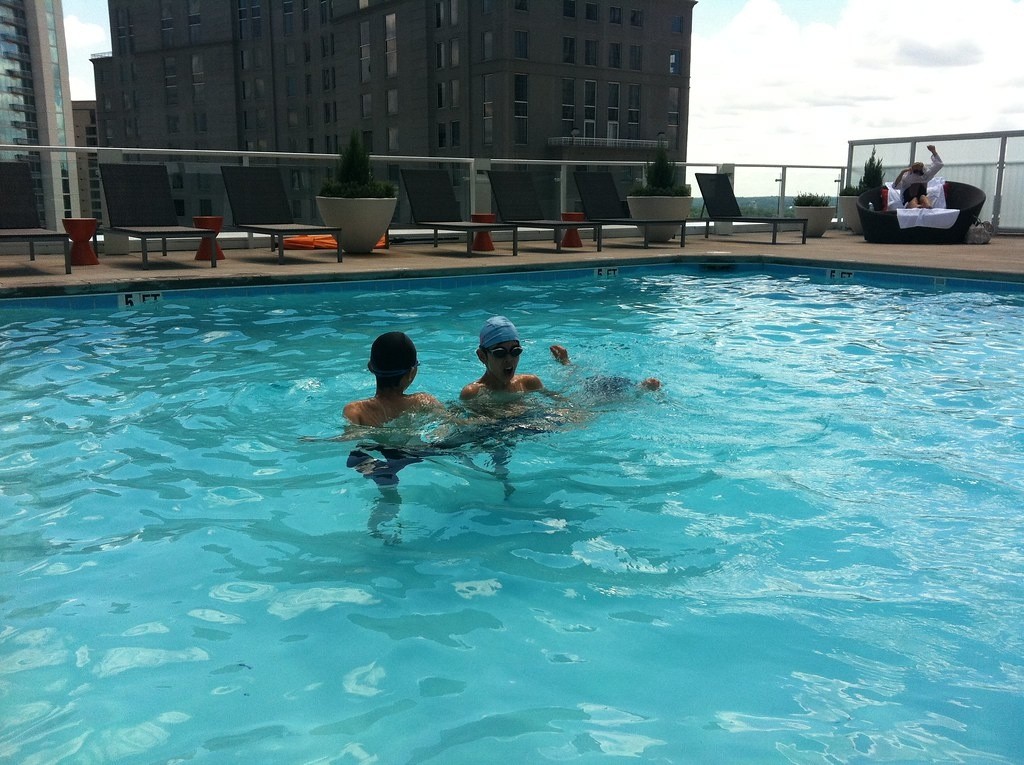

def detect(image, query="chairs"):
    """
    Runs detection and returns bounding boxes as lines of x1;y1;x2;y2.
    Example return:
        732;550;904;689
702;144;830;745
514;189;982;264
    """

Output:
98;163;218;269
0;161;72;274
485;170;602;253
220;165;343;265
695;173;808;244
401;169;518;258
574;171;686;248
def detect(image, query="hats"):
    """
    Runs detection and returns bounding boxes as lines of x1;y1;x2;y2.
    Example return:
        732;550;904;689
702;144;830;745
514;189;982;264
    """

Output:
369;331;418;370
480;316;519;348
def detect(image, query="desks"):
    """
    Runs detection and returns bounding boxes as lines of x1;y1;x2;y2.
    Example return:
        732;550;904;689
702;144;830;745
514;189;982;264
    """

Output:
192;217;226;261
61;218;100;264
470;213;494;251
561;212;585;247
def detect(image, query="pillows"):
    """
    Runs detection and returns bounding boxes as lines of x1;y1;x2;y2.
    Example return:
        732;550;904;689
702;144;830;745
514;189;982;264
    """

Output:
881;184;949;213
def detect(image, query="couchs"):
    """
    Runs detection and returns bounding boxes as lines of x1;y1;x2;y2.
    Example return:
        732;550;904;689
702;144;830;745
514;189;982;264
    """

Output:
856;179;986;244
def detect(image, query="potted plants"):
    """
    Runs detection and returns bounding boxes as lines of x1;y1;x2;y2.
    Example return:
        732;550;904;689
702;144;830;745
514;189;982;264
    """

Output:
838;144;885;235
627;152;694;242
316;129;399;253
794;193;835;238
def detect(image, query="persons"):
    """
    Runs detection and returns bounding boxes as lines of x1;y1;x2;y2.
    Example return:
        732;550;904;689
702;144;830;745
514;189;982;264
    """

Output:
459;316;663;399
343;332;451;427
892;145;944;208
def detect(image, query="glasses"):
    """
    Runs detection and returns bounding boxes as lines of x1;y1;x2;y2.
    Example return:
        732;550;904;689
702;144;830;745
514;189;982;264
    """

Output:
485;347;523;358
